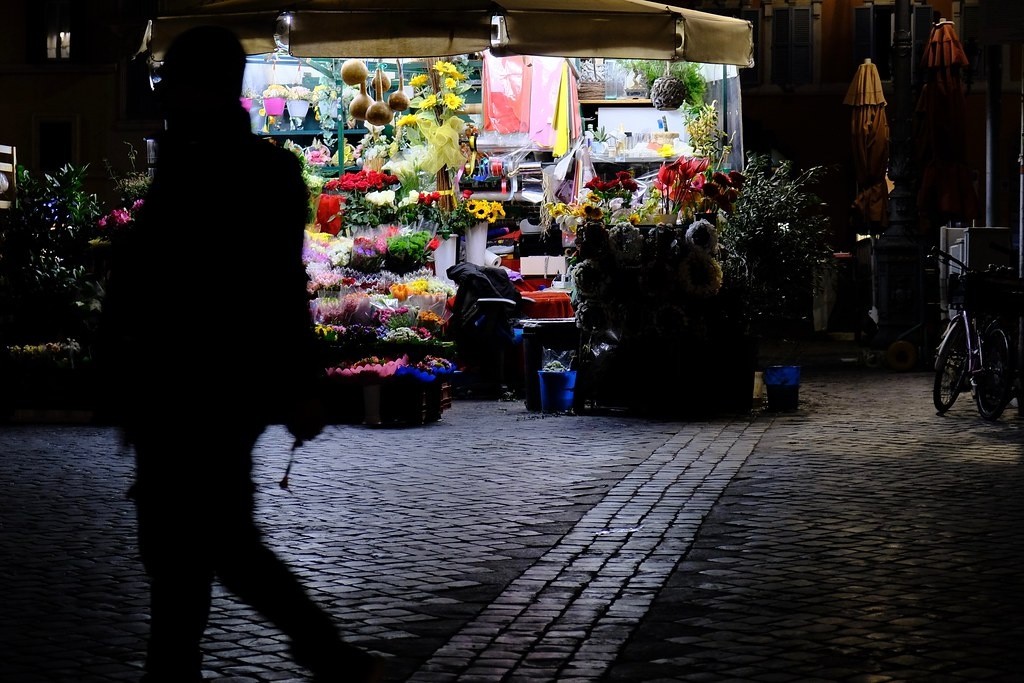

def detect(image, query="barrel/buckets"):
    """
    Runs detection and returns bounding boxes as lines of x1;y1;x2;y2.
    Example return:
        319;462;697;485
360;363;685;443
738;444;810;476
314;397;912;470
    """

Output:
537;369;576;413
763;364;800;412
753;372;763;398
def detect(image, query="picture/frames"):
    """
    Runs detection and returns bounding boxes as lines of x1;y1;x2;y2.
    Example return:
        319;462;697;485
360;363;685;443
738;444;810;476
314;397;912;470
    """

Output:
264;98;286;115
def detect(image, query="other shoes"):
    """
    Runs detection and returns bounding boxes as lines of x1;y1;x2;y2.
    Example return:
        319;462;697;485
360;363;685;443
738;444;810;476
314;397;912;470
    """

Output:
362;651;391;683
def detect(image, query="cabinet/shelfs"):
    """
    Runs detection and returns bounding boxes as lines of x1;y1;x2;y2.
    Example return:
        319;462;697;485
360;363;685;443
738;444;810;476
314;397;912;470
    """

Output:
245;57;483;178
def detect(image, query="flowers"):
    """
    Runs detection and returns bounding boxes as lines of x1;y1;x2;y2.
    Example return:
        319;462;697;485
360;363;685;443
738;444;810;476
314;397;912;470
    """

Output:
3;61;746;382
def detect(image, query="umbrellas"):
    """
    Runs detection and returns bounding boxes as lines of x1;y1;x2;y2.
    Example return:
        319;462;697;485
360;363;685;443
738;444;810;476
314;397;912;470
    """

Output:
917;17;975;233
842;57;892;309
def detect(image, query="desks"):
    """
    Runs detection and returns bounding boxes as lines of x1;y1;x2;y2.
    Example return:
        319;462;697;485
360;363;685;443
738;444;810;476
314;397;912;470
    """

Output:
448;291;575;319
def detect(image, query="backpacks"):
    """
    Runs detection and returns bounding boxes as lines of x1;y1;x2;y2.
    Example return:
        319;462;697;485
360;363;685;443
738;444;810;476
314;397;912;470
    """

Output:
214;134;323;440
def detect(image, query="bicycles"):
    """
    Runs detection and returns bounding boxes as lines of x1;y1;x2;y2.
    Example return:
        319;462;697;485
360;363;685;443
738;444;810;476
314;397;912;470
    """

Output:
925;244;1024;422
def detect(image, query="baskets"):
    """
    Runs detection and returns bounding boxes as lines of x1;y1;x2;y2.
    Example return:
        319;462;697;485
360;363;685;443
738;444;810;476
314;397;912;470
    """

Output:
946;273;982;311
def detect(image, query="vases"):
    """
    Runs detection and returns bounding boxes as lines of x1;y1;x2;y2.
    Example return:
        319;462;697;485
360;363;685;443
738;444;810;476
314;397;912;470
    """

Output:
692;212;718;227
651;213;678;224
287;100;309;117
319;101;337;117
240;97;252;112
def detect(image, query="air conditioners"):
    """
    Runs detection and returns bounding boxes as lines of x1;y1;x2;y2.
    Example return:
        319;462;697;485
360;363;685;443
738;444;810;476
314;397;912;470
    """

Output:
960;4;979;43
852;43;890;72
739;47;762;87
852;4;893;44
771;7;812;45
703;8;760;47
771;47;812;86
909;5;934;43
911;44;928;85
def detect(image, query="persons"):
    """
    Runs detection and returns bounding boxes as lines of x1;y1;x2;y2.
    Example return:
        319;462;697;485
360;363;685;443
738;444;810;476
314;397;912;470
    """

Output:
446;262;522;404
78;27;392;683
